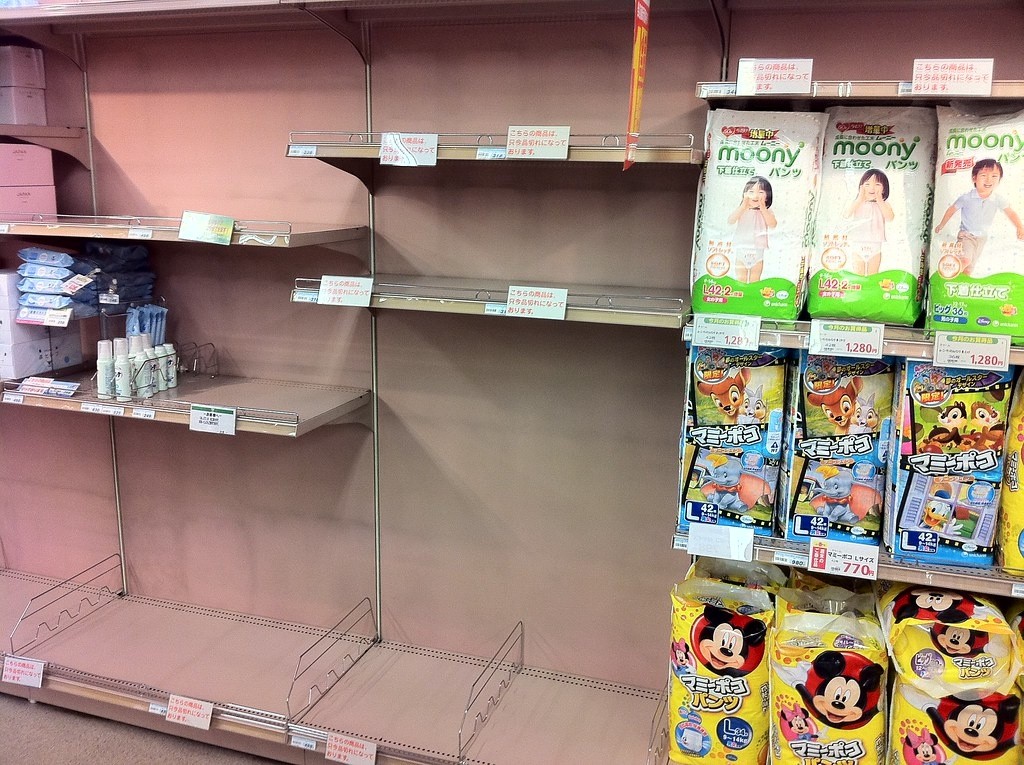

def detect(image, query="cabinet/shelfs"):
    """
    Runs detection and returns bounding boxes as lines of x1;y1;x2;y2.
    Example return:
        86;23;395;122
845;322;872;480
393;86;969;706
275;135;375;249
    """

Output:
0;2;1023;765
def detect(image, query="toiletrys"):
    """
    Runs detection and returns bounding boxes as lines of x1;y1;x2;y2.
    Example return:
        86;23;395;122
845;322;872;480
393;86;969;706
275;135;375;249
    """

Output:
96;332;177;402
